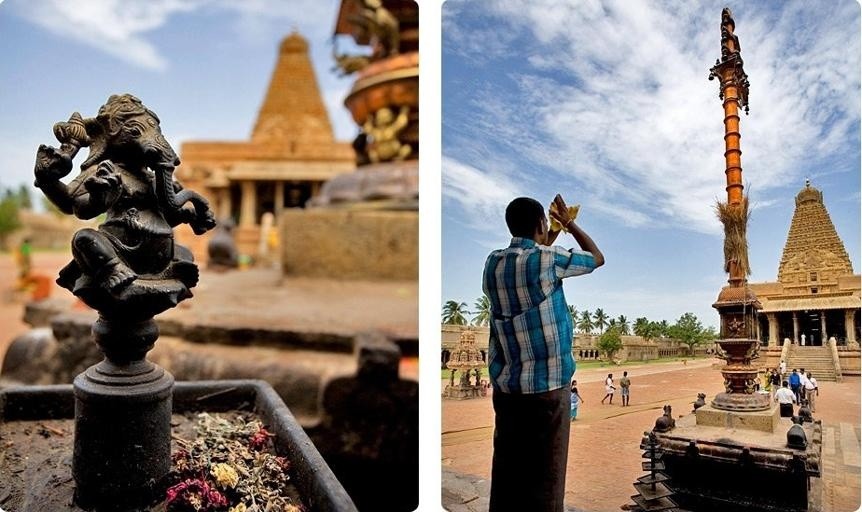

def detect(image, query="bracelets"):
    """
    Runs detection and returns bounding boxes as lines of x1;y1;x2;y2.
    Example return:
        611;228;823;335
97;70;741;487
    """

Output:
564;218;572;227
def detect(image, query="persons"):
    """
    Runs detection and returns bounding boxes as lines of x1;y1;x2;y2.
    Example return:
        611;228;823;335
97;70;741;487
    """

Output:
601;372;616;404
570;380;578;391
778;359;787;378
762;365;819;417
569;387;585;421
479;195;606;510
619;371;630;406
